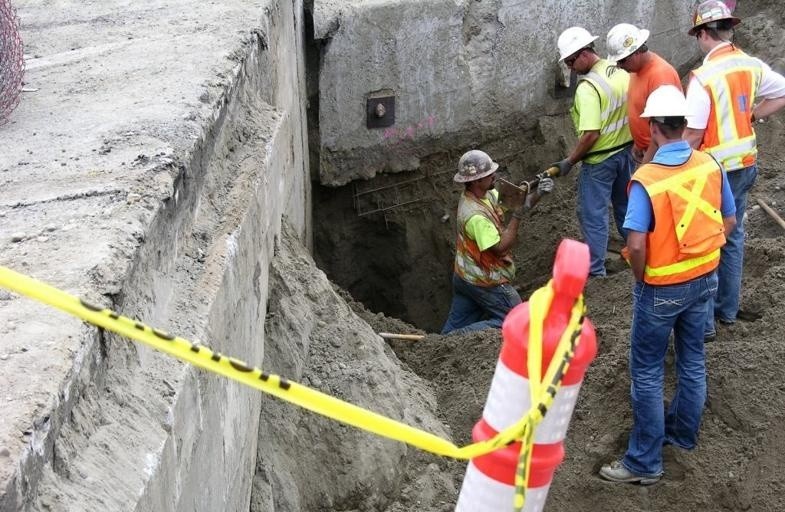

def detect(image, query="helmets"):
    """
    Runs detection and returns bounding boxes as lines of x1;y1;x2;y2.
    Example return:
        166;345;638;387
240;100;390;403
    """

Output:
688;0;742;36
557;26;600;63
639;85;695;118
454;150;499;183
606;23;650;63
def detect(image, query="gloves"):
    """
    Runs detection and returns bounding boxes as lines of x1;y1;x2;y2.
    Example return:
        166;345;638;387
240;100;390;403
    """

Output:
537;171;554;197
550;158;575;178
511;200;531;219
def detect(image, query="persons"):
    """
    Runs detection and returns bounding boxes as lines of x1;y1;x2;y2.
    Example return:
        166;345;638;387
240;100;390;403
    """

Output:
437;151;554;335
605;20;683;169
546;25;632;280
682;0;784;341
598;83;736;484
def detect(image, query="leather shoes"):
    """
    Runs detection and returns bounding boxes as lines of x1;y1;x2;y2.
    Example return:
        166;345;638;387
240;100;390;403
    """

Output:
599;462;661;485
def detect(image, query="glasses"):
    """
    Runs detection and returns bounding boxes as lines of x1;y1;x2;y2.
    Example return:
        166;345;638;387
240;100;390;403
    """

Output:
565;49;587;66
